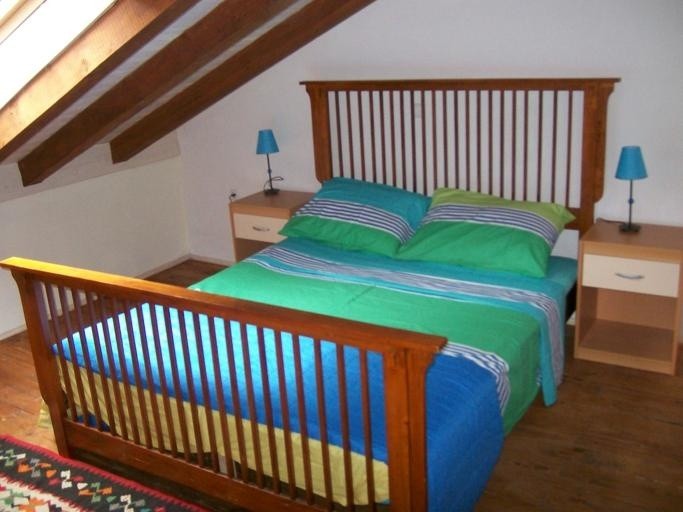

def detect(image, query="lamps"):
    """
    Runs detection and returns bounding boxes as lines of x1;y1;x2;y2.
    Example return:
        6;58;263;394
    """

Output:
256;129;280;194
615;146;647;233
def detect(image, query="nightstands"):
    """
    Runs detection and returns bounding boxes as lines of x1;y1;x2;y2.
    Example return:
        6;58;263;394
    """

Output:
573;220;683;376
228;191;314;263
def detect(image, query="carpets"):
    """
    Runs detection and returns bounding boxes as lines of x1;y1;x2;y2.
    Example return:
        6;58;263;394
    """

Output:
0;434;209;512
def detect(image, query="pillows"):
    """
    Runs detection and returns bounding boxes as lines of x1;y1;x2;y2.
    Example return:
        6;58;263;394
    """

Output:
278;177;576;277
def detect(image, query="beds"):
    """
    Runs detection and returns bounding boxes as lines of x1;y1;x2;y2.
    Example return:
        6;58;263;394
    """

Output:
0;78;621;512
